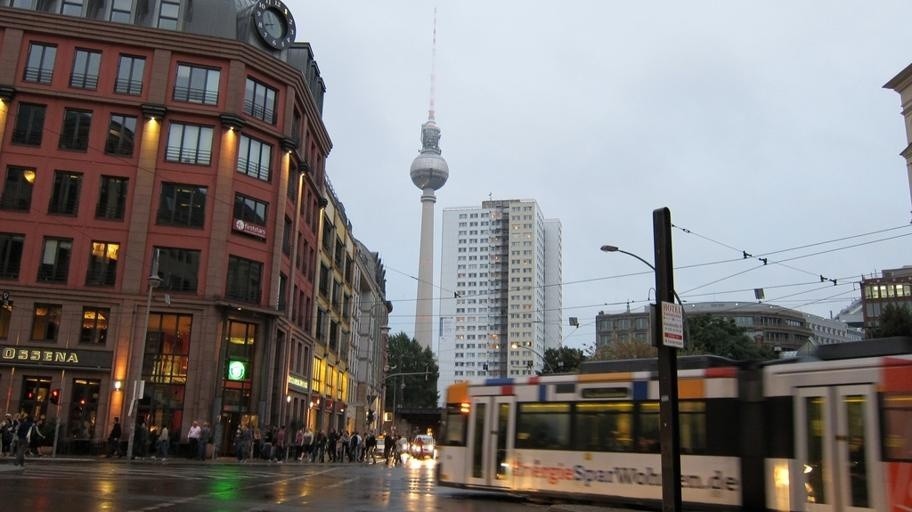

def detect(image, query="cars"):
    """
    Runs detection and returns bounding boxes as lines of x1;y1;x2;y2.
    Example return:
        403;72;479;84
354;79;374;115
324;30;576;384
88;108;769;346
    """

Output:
371;434;436;457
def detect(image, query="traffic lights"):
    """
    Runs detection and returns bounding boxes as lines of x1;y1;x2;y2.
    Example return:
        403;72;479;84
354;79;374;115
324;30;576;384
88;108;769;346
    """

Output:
50;388;58;405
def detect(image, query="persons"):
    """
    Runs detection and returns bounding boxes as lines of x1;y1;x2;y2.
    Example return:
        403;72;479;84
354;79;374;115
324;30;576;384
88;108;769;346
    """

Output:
0;413;46;468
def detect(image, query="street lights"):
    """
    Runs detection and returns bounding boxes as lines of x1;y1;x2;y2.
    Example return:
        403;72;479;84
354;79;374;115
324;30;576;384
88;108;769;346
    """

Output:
511;344;555;375
126;249;164;461
600;245;692;357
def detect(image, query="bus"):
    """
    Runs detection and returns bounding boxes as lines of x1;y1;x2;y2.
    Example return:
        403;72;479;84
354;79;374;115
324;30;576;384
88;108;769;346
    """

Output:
435;334;910;511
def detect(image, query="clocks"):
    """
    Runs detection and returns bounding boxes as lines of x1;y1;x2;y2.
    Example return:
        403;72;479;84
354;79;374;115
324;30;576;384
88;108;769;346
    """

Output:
251;0;296;51
